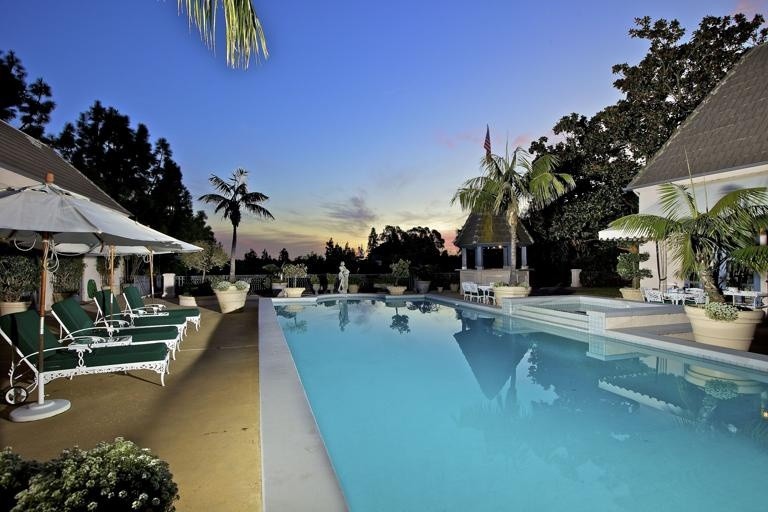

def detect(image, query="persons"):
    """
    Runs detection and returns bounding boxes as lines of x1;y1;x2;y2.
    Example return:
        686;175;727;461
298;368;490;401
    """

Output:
337;260;350;294
338;301;350;332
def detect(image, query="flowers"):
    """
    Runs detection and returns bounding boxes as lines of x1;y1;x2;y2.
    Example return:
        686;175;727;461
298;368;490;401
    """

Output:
196;167;275;291
608;180;767;321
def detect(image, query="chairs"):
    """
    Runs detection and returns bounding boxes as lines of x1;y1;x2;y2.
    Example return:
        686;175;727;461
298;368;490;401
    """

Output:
462;280;497;306
644;283;768;311
0;286;200;405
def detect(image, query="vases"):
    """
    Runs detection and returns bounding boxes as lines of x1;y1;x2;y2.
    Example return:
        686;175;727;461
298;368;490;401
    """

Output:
213;287;250;314
496;286;532;299
683;304;765;351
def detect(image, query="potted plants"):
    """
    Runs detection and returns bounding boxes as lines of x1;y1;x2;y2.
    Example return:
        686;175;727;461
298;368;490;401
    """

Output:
385;302;411;334
448;138;576;290
261;258;459;314
284;306;309;335
617;252;653;301
